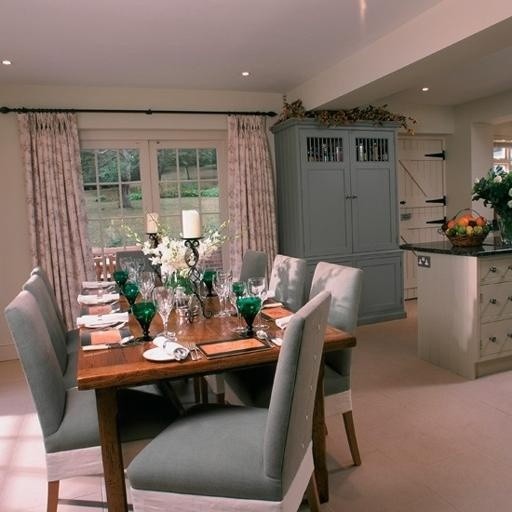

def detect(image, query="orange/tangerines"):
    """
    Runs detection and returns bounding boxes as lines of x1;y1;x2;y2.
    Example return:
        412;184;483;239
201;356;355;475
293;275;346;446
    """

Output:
447;228;456;235
482;225;491;234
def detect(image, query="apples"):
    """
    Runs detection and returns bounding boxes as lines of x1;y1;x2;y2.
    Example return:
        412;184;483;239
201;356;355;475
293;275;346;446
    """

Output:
448;221;456;229
476;216;487;228
458;216;469;227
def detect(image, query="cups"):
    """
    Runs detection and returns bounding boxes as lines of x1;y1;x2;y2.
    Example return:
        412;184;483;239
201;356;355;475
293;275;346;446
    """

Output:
137;272;155;302
152;286;176;343
213;269;270;332
119;257;139;283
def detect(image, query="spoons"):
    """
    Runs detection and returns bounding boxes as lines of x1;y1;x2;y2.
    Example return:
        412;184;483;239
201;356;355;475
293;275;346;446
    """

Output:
256;329;275;348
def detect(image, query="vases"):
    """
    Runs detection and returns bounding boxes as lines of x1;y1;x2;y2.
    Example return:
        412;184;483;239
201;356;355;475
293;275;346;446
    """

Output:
495;211;511;245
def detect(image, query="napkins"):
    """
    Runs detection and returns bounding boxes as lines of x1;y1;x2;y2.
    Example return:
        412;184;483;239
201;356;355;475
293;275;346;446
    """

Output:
76;293;119;305
76;312;129;326
152;336;189;362
274;315;295;329
81;280;117;289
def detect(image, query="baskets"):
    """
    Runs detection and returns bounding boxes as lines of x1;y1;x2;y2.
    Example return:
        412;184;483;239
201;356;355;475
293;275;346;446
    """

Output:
441;208;492;246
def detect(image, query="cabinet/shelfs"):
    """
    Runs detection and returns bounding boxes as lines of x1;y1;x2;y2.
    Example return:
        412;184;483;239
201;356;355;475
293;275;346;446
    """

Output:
269;117;408;327
479;253;512;357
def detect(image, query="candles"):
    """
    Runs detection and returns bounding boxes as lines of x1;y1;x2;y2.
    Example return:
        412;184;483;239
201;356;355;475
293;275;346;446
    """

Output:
181;209;201;238
146;212;159;232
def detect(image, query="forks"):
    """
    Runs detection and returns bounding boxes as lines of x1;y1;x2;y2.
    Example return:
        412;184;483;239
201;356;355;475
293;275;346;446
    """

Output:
184;342;200;360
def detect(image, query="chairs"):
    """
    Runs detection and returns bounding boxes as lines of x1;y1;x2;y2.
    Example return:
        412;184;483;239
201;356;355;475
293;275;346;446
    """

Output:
20;277;167;395
3;291;174;511
232;262;363;504
126;289;330;511
236;249;270;297
216;255;307;403
30;267;81;355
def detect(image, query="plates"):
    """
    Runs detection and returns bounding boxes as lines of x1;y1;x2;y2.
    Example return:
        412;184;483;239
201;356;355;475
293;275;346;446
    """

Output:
82;282;109;289
141;346;180;361
84;314;118;329
78;294;112;305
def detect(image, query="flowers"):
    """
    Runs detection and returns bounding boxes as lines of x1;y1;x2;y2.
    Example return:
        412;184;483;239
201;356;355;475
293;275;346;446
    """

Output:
118;205;231;283
469;165;511;247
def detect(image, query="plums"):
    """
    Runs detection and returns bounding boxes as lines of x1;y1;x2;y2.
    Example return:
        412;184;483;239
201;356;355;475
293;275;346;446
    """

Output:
469;221;476;228
